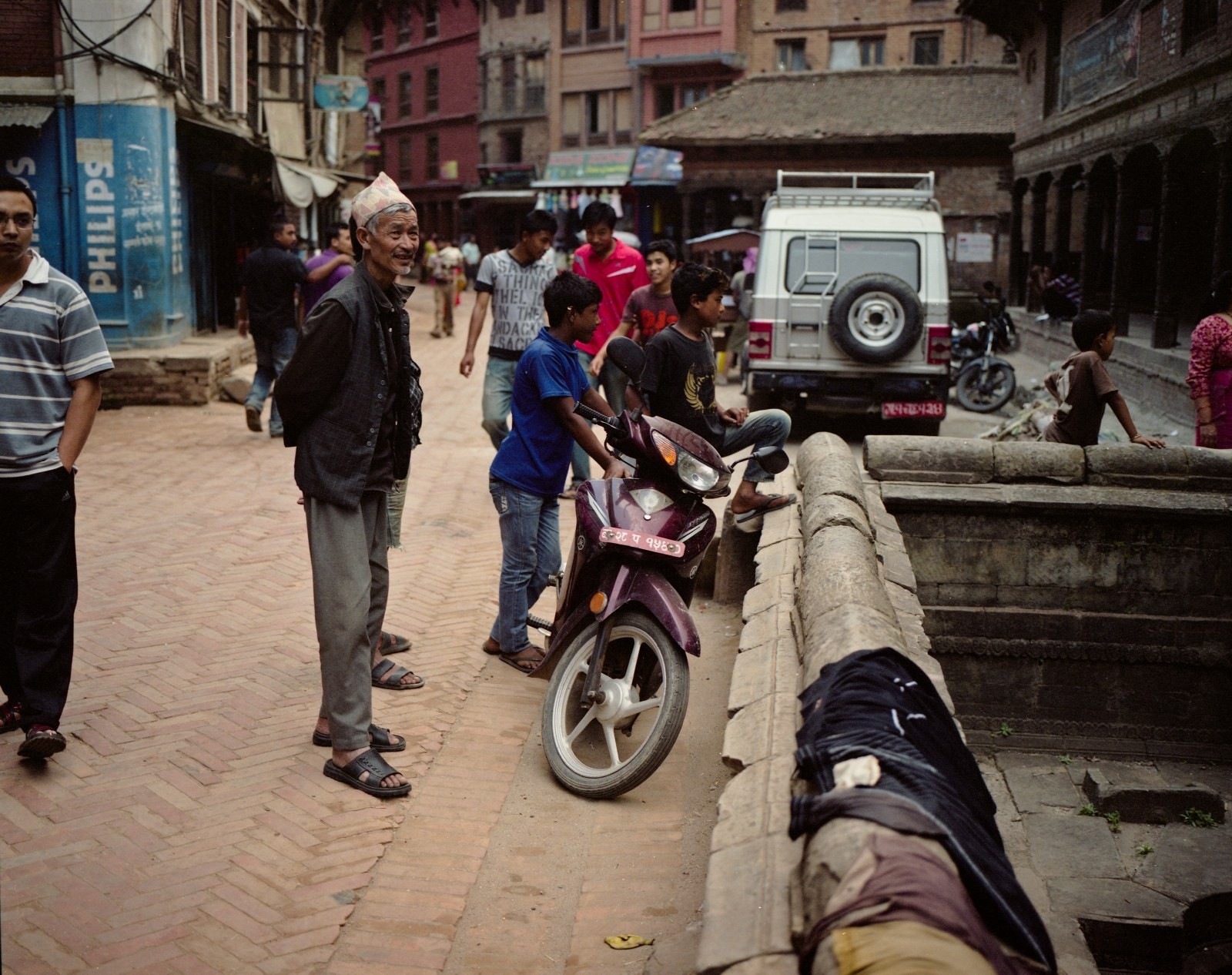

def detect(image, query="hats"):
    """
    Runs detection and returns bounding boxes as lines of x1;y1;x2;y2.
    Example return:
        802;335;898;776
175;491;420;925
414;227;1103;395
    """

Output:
350;170;416;225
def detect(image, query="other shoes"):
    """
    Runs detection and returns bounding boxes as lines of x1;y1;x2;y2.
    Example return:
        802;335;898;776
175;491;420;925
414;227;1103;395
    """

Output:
247;405;263;433
431;333;442;338
560;488;577;499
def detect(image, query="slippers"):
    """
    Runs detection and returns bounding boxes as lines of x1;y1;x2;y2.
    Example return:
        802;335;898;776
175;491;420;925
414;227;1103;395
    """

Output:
481;640;502;655
736;493;797;523
376;633;411;655
16;726;66;761
0;700;24;732
313;722;405;752
499;647;542;674
324;748;411;796
367;659;426;690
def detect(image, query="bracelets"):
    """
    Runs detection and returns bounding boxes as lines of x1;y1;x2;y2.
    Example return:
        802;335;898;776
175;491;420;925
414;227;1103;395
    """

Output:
1200;420;1213;426
1130;433;1142;440
1197;404;1211;411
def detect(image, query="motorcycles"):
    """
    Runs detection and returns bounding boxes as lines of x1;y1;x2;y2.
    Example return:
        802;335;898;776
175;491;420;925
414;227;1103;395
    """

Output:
949;281;1016;414
525;335;789;802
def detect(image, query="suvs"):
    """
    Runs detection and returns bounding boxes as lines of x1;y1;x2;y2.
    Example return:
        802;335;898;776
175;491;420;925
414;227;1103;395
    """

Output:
744;170;954;437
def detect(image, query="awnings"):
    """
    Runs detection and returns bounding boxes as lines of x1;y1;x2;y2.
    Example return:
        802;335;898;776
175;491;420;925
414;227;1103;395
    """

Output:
459;191;537;199
0;104;55;128
180;115;379;208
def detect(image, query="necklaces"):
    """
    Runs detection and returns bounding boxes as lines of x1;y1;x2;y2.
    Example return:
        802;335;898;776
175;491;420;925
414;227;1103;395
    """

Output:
1225;311;1232;314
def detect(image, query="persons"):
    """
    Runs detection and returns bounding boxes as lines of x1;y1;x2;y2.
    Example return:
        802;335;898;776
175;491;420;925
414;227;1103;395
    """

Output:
641;264;798;524
1036;298;1166;449
1025;259;1082;324
269;172;424;799
239;202;762;441
1186;270;1232;450
483;271;629;680
0;170;114;761
